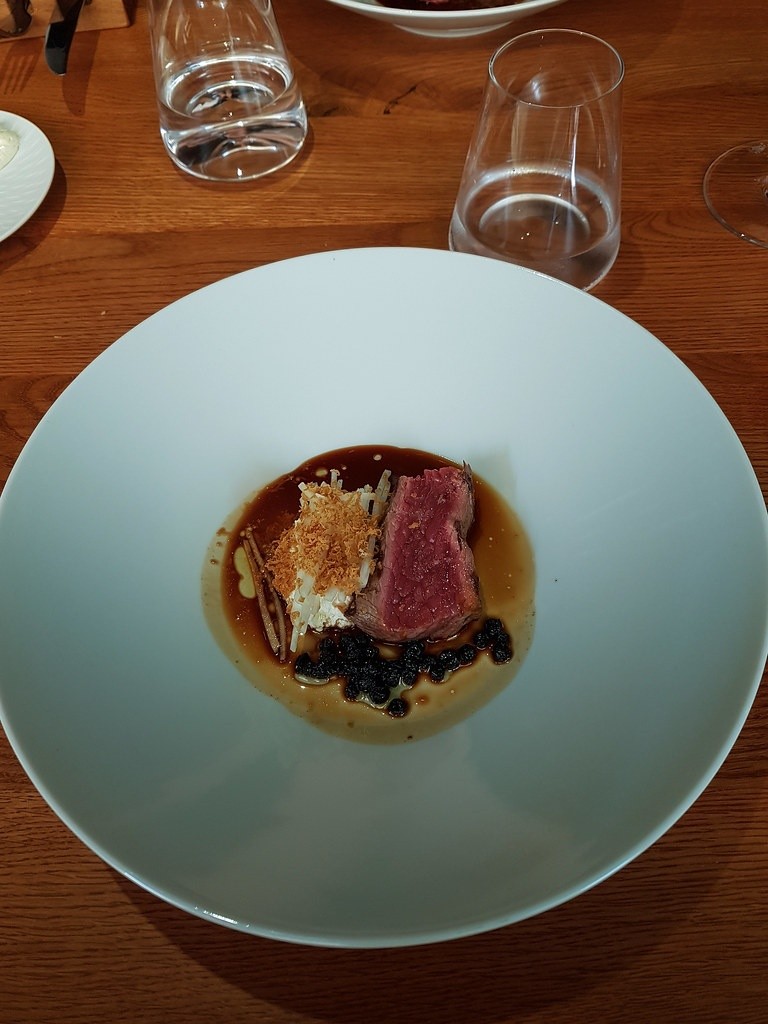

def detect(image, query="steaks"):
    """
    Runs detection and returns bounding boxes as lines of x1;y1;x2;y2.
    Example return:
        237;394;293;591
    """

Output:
353;461;481;644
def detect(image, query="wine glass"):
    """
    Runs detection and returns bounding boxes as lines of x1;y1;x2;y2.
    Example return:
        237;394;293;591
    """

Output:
702;138;768;248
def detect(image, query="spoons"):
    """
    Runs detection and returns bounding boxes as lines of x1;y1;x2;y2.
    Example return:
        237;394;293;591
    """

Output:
0;0;33;38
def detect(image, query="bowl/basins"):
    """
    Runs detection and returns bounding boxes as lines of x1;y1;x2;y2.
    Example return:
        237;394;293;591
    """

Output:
327;0;567;39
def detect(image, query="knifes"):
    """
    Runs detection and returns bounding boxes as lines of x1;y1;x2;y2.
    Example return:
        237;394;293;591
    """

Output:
43;0;86;77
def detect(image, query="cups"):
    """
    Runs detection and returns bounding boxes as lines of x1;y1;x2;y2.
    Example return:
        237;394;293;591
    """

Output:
145;0;308;183
446;28;625;292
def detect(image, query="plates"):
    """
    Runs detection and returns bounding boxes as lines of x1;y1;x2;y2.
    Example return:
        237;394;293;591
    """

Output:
0;109;56;242
0;246;766;949
